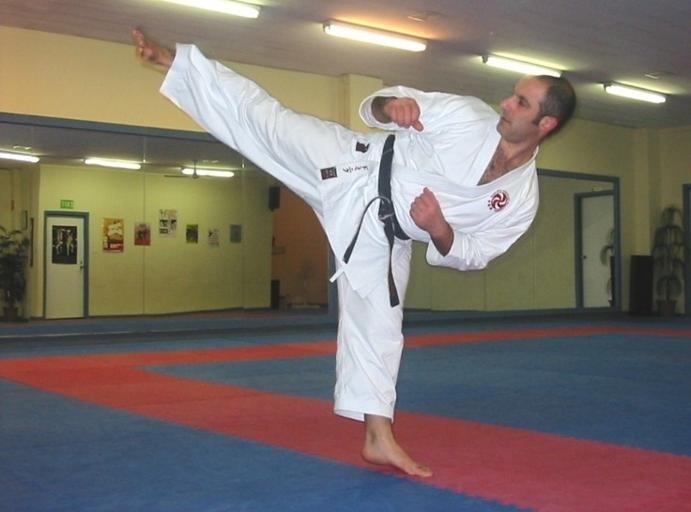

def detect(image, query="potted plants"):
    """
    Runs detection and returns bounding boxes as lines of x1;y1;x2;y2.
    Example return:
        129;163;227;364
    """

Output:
650;206;688;322
0;225;32;321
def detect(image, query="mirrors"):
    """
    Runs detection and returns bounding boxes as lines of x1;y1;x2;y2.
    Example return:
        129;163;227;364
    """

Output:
1;109;623;340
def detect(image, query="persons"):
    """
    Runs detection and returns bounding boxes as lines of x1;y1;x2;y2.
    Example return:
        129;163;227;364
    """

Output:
131;27;577;478
67;232;75;256
56;231;67;256
